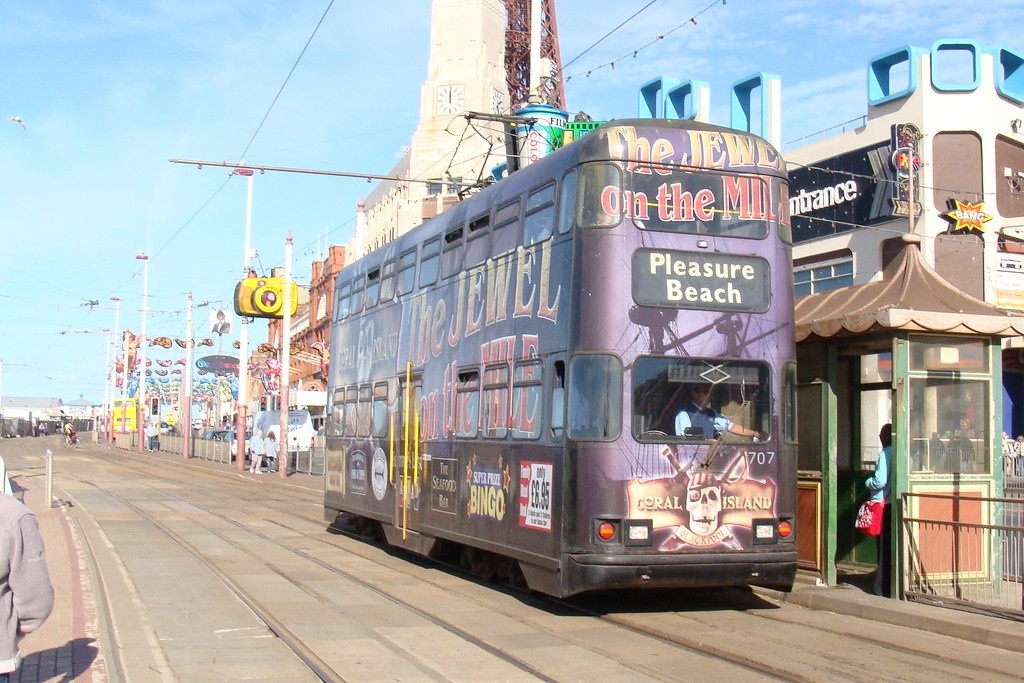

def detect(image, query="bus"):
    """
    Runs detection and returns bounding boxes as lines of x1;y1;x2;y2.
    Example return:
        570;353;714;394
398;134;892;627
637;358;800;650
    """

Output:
314;110;803;611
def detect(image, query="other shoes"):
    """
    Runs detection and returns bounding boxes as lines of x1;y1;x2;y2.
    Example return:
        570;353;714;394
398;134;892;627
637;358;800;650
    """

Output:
255;470;262;474
271;469;275;473
249;467;254;473
268;470;271;474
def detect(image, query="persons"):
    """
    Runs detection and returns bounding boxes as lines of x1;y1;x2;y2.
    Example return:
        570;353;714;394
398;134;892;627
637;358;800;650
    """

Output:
212;311;230;333
39;422;48;436
263;431;279;473
674;382;768;441
0;456;54;683
1002;432;1011;475
864;424;892;595
929;430;975;470
1014;435;1024;476
317;429;324;437
146;421;157;452
250;429;264;474
65;421;77;443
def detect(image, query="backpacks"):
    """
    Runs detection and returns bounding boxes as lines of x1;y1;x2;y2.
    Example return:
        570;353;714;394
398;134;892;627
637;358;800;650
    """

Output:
66;423;77;436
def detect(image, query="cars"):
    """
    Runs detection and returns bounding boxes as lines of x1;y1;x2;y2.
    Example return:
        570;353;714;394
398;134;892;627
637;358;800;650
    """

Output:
202;410;318;473
161;422;174;435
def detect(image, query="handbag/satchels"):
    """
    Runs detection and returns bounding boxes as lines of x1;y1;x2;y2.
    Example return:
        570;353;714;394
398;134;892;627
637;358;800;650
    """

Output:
856;486;892;537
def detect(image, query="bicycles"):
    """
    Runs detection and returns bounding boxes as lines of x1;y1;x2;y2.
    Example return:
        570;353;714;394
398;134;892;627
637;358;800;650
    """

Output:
61;431;80;450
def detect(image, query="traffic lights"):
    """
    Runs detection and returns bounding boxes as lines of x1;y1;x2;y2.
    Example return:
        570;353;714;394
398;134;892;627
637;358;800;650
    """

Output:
260;396;268;412
151;397;159;416
275;395;281;411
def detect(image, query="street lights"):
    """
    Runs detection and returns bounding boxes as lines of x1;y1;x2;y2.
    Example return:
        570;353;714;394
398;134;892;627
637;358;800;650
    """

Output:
109;295;120;448
134;250;148;453
234;167;254;471
